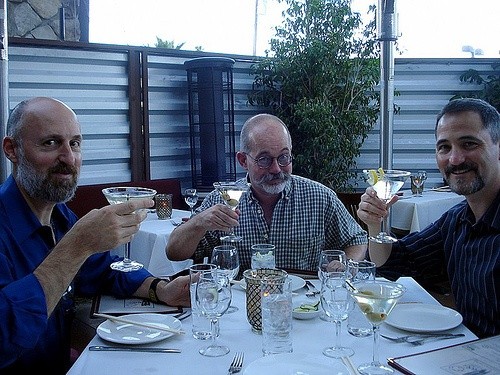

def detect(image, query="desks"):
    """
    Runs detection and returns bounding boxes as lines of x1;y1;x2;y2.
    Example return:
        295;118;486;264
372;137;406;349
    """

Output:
109;209;198;281
64;277;480;375
389;188;466;239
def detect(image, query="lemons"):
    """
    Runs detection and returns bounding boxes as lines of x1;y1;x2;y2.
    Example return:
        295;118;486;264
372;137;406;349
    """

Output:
367;169;384;186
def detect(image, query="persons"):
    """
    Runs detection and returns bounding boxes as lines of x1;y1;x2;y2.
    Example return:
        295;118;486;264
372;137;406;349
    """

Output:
356;99;500;340
165;114;368;272
0;97;201;375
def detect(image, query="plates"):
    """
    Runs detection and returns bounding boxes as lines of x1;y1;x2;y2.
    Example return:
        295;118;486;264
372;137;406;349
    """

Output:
291;310;323;320
239;274;306;291
384;303;463;333
243;354;352;375
96;313;184;345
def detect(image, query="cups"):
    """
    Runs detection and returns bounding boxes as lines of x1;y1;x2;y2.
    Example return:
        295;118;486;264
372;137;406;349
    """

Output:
251;243;276;269
189;264;220;340
347;261;377;337
155;194;173;220
260;277;294;358
243;269;289;336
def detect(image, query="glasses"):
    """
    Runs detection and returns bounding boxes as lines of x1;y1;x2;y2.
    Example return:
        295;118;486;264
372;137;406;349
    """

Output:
246;152;295;169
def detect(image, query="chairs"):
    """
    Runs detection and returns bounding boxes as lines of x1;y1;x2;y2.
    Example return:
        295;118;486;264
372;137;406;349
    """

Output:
334;193;368;232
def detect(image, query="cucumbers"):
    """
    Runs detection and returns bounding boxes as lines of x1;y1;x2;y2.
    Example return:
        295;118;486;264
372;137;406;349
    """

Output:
293;301;320;312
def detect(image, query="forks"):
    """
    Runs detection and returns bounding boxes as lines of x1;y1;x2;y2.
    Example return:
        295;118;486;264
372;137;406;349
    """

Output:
228;352;244;375
380;334;465;343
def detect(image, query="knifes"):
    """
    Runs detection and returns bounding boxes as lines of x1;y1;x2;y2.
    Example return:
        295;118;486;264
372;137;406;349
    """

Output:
89;345;182;353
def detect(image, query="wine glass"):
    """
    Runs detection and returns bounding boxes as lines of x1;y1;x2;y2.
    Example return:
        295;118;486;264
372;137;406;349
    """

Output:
363;170;411;244
184;189;199;215
347;279;408;375
102;187;157;273
317;250;347;301
210;245;240;314
321;272;356;359
196;272;232;357
412;173;424;197
213;181;251;242
418;171;427;195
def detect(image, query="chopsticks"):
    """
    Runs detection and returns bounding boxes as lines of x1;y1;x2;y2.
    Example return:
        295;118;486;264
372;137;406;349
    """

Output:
93;313;186;335
342;356;361;375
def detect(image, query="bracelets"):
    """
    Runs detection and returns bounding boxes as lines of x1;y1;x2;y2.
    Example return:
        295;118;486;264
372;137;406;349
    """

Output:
149;278;171;304
